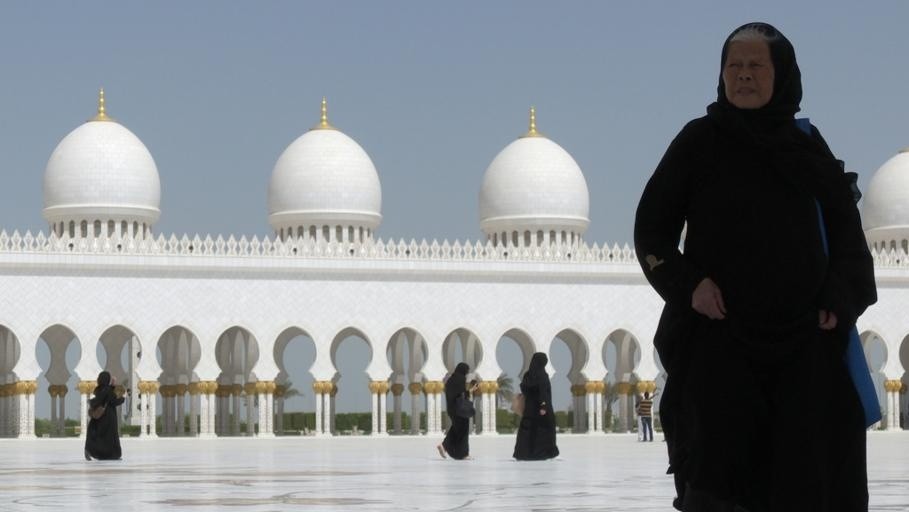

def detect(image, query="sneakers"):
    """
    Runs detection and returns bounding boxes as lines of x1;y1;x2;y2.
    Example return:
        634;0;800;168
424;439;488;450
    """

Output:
437;443;448;458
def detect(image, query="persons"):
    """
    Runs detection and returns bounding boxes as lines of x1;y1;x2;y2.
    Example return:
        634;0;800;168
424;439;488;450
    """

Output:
437;362;479;460
85;370;124;461
636;391;654;441
634;22;877;512
512;352;559;461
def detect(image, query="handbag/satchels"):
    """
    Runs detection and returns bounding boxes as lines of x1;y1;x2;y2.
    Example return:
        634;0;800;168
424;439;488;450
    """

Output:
88;405;106;419
511;393;527;416
846;327;882;428
454;396;476;418
